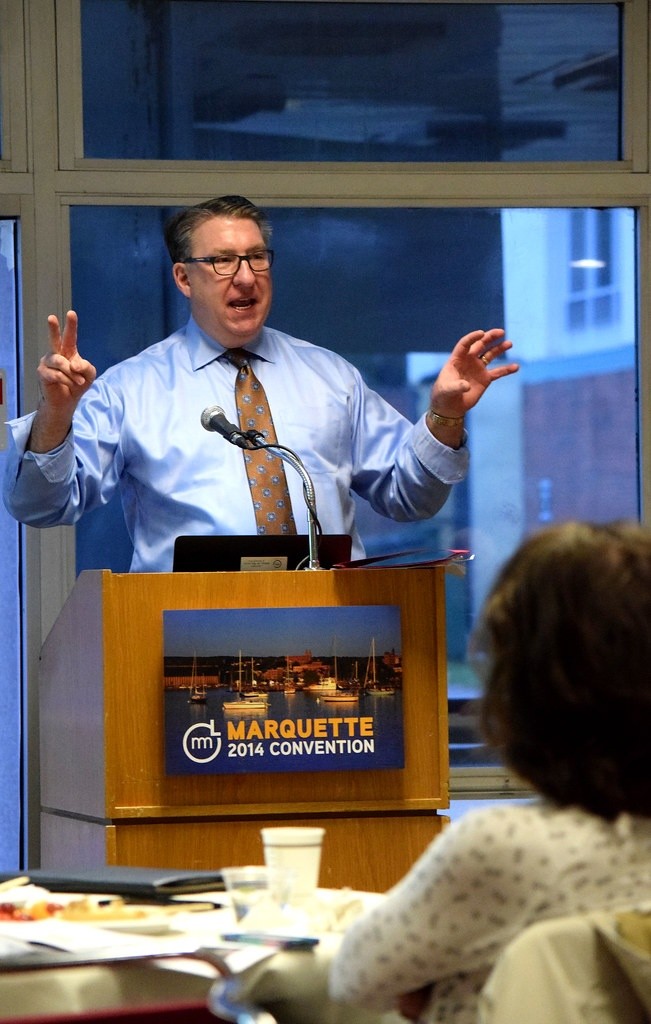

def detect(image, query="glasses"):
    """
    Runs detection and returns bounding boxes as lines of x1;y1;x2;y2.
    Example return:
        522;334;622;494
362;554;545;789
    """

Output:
178;248;274;276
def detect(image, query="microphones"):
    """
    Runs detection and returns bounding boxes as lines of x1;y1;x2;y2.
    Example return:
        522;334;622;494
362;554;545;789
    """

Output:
200;406;250;450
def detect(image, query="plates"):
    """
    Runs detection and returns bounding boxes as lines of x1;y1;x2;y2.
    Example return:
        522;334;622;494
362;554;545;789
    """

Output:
52;905;191;935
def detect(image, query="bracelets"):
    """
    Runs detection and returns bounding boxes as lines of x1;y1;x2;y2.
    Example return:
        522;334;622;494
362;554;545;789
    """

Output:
428;408;465;427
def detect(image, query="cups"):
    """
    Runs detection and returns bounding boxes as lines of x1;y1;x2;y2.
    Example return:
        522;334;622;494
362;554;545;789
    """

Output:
261;827;327;907
220;865;299;932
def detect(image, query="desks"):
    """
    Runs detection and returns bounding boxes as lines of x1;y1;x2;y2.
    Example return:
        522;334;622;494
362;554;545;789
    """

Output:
0;889;434;1024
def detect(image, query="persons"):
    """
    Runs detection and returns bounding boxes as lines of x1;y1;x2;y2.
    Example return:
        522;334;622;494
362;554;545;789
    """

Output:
4;194;519;574
327;522;650;1020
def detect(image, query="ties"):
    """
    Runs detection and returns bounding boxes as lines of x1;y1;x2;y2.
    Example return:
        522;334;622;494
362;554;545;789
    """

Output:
223;349;298;536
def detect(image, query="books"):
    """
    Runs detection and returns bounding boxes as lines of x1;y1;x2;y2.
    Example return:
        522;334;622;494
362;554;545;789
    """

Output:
1;865;227;897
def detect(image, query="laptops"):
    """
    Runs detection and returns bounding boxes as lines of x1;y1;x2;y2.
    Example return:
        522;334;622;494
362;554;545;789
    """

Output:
172;533;352;573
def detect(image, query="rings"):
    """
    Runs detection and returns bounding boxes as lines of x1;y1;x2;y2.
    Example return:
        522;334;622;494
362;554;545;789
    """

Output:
481;356;490;365
